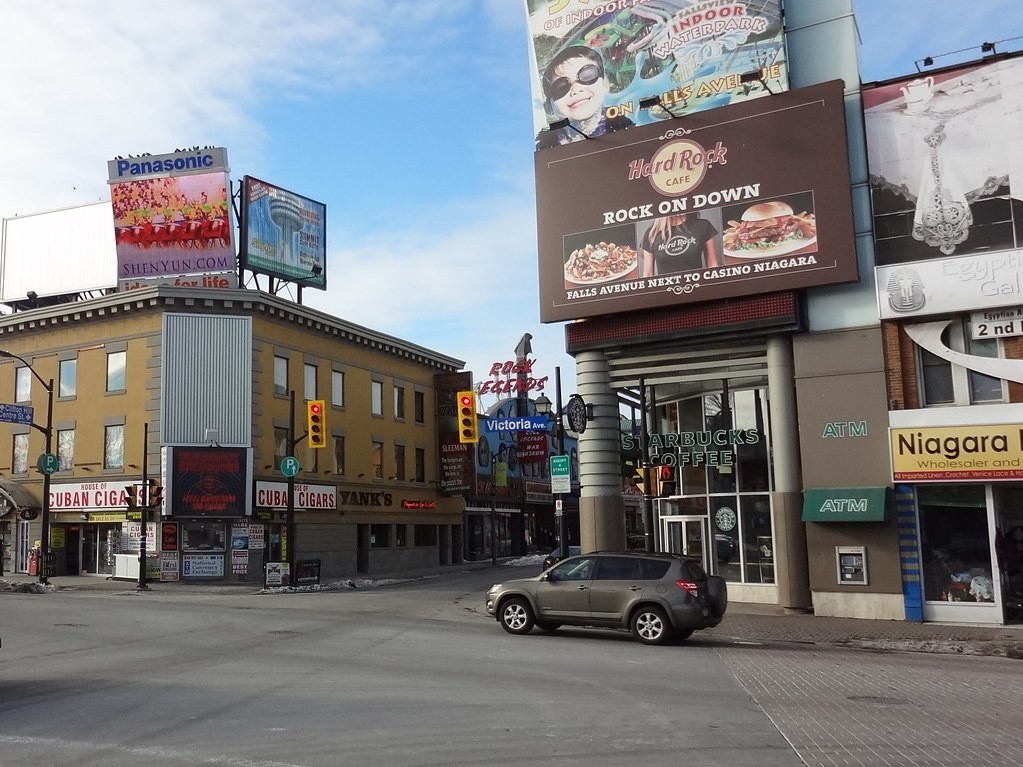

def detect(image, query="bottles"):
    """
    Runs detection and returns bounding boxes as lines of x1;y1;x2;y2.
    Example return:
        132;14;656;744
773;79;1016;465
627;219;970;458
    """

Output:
947;587;953;601
941;586;947;601
959;588;969;601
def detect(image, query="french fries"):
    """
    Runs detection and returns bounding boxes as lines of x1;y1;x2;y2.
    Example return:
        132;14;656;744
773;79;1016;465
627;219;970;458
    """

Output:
723;211;815;250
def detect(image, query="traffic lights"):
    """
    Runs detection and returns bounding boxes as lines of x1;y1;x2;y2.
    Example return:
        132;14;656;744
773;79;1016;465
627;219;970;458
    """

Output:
148;479;164;506
655;463;677;499
457;392;479;444
308;399;328;448
123;485;138;506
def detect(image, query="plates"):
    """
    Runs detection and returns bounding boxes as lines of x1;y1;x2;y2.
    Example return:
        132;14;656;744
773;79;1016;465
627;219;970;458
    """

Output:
722;219;819;259
564;249;640;284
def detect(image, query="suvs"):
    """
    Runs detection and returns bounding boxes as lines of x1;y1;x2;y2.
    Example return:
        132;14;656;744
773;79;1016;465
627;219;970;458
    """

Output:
485;547;728;647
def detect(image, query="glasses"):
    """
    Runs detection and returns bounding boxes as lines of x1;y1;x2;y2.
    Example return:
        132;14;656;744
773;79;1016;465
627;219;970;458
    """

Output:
547;64;604;103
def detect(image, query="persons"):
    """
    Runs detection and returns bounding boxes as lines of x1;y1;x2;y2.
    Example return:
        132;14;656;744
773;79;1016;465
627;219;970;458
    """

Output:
639;207;721;278
126;188;228;226
535;45;635;151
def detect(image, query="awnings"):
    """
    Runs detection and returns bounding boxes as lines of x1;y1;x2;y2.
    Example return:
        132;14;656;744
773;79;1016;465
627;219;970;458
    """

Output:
801;485;892;522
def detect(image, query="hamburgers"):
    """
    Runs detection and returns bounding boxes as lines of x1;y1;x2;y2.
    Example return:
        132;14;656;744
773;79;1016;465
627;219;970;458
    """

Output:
737;201;800;243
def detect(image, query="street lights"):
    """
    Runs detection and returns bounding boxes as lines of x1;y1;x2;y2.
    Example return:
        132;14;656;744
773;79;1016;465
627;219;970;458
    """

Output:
0;349;56;582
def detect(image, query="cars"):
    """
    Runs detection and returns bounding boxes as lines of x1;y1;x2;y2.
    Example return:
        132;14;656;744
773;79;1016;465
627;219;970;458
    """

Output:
542;546;583;574
